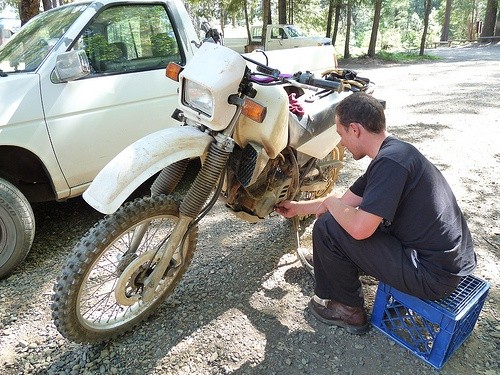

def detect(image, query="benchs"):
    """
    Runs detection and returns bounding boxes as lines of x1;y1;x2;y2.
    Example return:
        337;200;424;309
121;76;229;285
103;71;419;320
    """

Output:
433;36;500;48
99;31;181;70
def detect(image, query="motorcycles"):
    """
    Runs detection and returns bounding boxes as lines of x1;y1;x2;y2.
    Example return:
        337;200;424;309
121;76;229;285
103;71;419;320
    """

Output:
48;21;354;347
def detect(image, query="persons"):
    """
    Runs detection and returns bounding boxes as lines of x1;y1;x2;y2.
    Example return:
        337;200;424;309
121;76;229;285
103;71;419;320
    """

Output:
275;91;477;334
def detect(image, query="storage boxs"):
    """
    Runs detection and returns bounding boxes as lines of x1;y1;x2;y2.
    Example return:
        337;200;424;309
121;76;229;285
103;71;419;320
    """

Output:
370;273;491;372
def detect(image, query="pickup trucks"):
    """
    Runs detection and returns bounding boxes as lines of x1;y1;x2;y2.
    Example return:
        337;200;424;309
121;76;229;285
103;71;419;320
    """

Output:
223;24;333;53
0;0;340;281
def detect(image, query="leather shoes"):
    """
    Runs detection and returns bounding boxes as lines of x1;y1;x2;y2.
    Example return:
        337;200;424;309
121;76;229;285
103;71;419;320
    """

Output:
308;294;369;334
